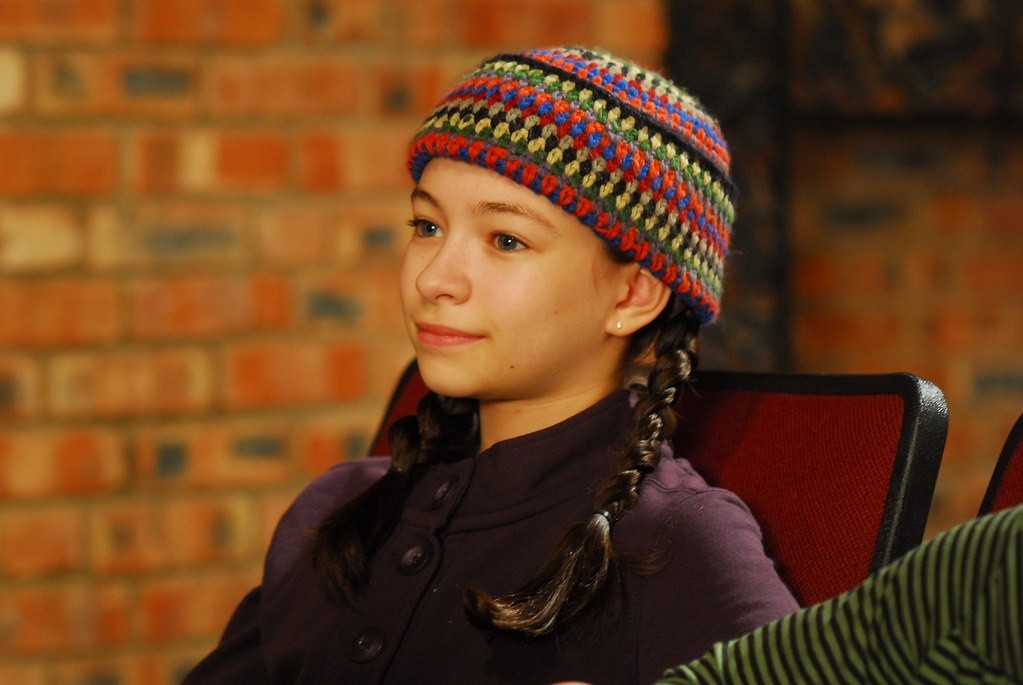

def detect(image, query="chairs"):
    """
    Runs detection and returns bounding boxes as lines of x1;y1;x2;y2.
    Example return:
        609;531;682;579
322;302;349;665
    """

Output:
351;352;949;609
973;412;1022;516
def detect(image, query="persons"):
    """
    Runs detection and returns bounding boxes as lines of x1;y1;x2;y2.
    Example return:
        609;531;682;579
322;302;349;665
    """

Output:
657;504;1022;684
180;44;807;684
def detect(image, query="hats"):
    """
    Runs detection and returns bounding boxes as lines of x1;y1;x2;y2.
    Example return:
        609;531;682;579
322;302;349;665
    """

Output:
407;45;738;325
